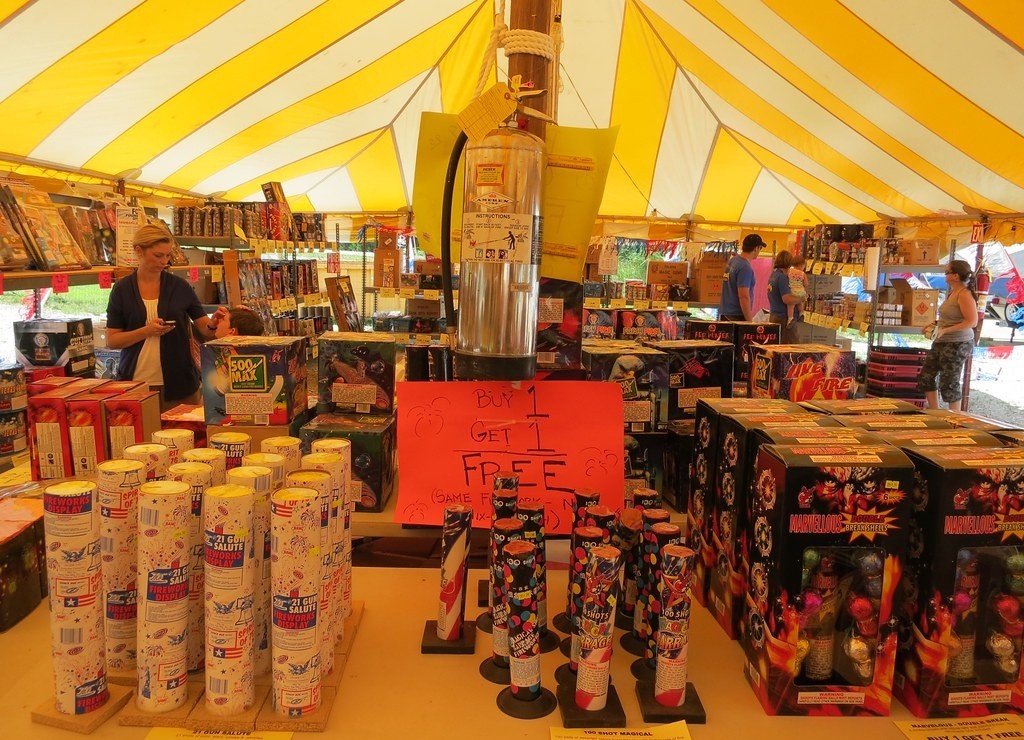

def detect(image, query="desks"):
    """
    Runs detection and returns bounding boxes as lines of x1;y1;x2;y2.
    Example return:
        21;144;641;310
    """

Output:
0;565;1024;739
0;498;48;600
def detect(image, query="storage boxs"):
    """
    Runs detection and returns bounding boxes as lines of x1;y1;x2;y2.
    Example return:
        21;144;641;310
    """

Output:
623;399;1023;717
0;184;458;514
537;236;940;433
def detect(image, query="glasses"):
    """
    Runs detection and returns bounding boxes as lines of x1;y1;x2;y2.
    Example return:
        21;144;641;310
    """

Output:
945;270;953;275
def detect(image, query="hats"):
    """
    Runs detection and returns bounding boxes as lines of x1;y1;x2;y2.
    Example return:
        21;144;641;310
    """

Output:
743;234;767;248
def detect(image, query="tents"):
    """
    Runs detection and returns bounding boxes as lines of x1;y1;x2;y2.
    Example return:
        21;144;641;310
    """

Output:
2;0;1024;261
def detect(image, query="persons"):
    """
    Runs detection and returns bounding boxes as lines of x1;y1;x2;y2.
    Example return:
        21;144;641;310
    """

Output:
215;307;264;340
917;259;978;412
782;255;808;329
718;234;767;323
768;249;802;344
104;225;227;416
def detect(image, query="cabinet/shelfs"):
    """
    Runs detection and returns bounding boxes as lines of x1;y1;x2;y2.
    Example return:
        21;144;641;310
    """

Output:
166;210;340;315
361;225;458;333
772;235;956;397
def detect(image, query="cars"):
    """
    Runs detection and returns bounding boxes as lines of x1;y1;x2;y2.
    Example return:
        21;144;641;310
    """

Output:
920;273;949;311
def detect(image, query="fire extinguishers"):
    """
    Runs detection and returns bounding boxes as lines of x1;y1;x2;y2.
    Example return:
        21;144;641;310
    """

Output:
440;73;559;381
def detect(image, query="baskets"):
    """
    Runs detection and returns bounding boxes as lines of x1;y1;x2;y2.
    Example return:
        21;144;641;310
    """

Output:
865;345;930;410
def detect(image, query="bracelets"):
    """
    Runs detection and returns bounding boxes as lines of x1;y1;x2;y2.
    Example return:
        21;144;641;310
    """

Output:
932;320;937;326
207;322;218;330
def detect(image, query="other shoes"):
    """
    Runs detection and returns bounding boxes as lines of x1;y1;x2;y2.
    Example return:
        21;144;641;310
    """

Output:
797;314;804;323
786;317;794;329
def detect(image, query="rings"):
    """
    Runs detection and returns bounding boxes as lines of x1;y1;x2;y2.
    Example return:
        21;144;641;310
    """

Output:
159;331;162;336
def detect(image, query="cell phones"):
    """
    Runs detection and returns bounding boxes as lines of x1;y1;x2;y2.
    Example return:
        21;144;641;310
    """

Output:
162;321;176;326
